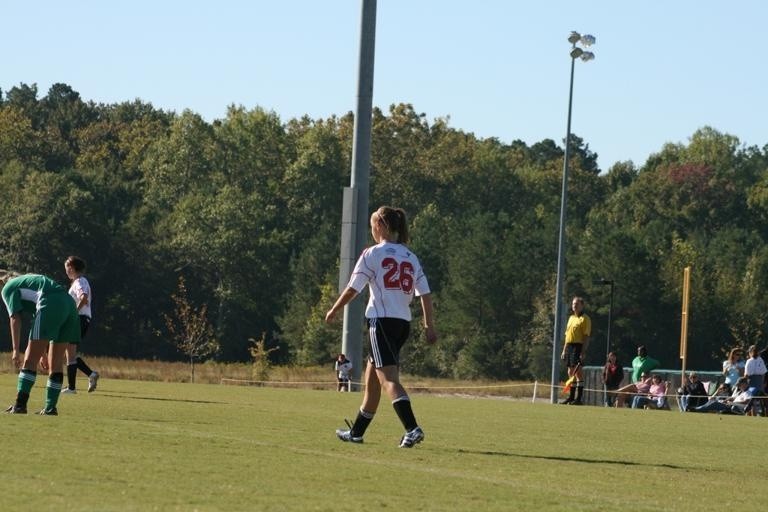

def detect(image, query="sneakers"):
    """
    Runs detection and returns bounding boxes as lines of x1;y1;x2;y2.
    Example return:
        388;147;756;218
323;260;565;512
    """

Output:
35;409;58;415
3;404;28;414
336;429;364;444
60;386;76;394
398;427;424;448
571;399;582;405
88;372;99;392
559;397;574;404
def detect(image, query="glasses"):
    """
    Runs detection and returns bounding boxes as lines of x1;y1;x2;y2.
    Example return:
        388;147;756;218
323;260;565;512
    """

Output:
735;353;741;357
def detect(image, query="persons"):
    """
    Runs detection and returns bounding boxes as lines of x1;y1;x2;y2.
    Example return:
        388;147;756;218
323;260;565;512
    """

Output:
335;354;352;392
61;254;99;394
0;269;82;416
601;344;767;418
326;205;436;447
558;296;591;405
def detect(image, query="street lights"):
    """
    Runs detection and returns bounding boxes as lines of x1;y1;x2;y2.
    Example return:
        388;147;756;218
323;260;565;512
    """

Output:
548;27;596;403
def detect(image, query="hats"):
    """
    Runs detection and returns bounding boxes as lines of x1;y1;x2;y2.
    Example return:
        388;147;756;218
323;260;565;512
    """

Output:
734;377;747;386
